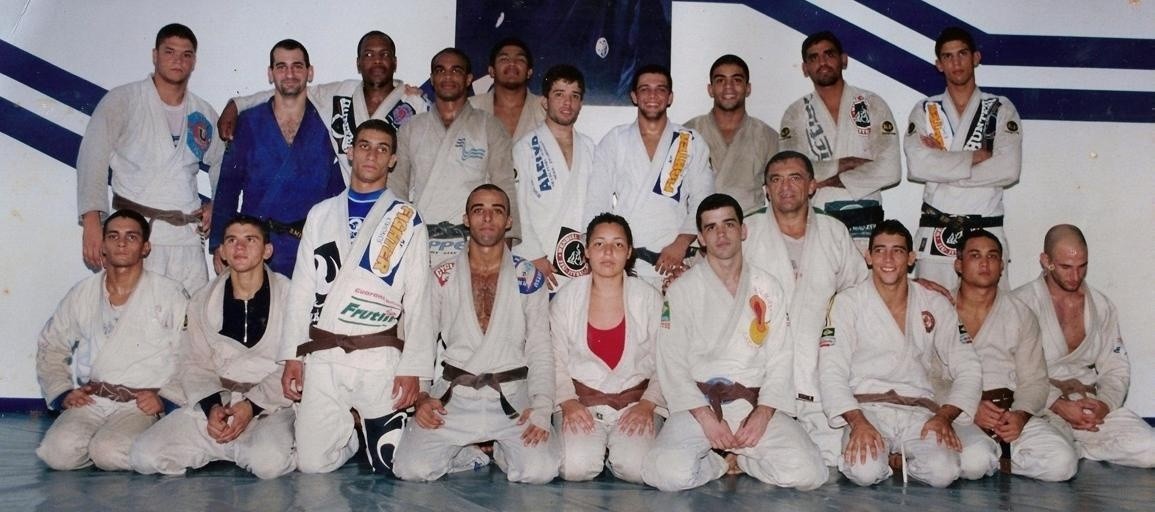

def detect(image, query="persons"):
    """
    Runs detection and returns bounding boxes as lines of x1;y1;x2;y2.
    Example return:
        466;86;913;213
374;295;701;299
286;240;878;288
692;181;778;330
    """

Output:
814;219;987;491
660;149;958;472
388;183;569;485
128;216;299;481
942;228;1081;485
271;120;439;476
548;210;666;485
641;191;831;495
30;209;193;471
1009;219;1155;485
71;21;226;297
